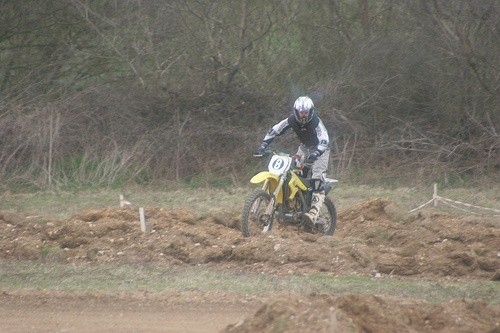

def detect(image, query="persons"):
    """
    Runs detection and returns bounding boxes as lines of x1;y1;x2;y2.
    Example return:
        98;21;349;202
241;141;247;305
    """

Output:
255;96;330;233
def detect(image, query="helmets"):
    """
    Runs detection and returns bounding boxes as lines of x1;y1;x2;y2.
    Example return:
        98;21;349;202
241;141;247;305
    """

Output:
293;96;315;124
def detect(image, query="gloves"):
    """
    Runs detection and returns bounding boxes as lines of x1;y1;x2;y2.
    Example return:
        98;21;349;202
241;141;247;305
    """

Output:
255;140;267;154
307;151;320;162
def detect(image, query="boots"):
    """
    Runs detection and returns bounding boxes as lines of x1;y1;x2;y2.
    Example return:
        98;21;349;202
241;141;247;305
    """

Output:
303;193;325;232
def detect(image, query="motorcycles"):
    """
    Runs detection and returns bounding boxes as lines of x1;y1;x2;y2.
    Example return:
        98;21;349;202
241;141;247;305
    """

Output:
240;148;339;240
